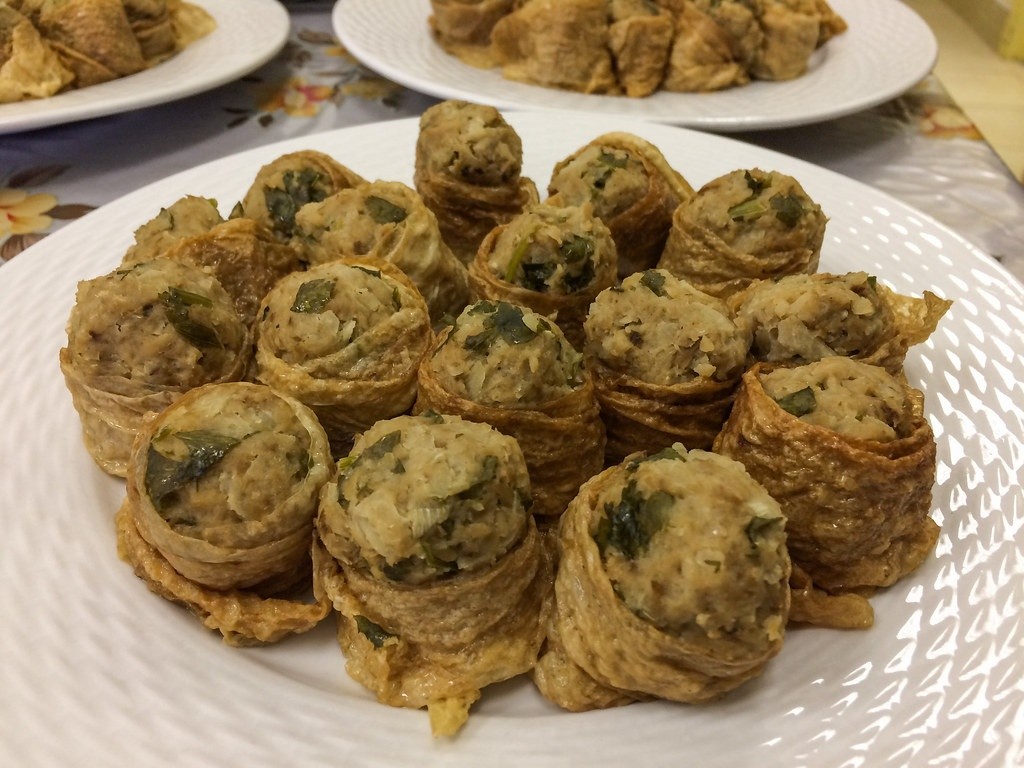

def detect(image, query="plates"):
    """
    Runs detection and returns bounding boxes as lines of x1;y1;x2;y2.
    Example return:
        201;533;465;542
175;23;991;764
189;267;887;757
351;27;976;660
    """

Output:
0;0;291;133
330;0;939;132
0;109;1024;768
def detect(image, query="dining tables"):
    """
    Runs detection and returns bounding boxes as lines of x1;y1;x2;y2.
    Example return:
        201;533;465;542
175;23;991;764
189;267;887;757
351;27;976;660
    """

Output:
2;1;1021;768
